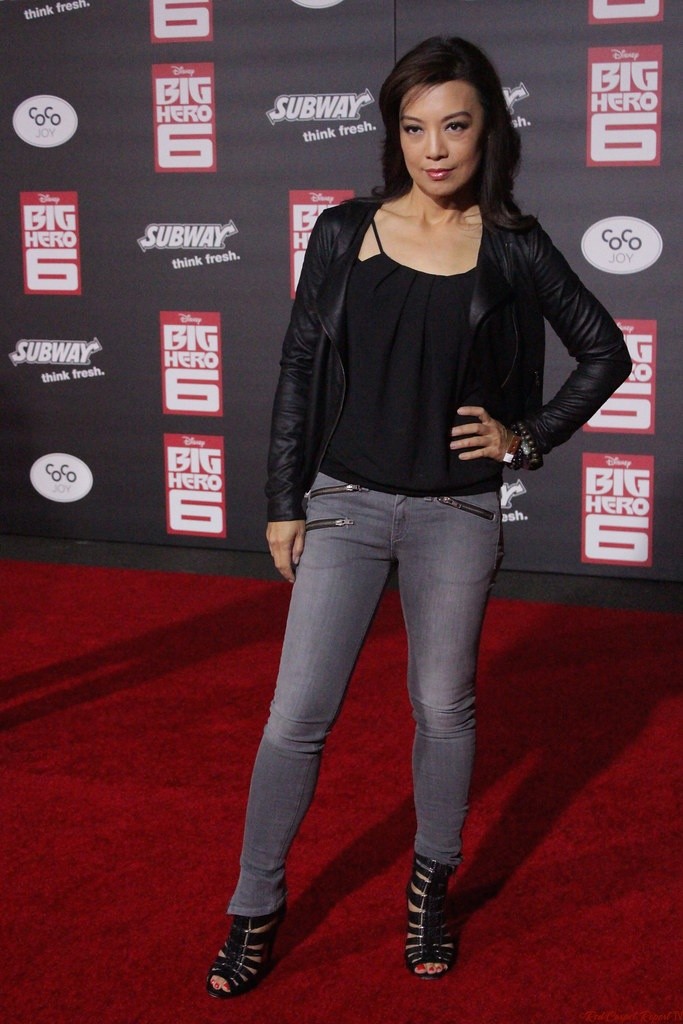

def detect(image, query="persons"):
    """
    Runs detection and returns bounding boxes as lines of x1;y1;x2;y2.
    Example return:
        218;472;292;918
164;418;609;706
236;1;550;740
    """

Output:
205;40;634;998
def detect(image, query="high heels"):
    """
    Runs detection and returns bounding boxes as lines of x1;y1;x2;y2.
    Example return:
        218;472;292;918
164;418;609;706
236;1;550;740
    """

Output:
204;900;290;997
404;853;459;981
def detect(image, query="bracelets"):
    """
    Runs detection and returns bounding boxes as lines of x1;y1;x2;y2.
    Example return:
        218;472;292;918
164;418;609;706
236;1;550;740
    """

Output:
502;421;543;470
